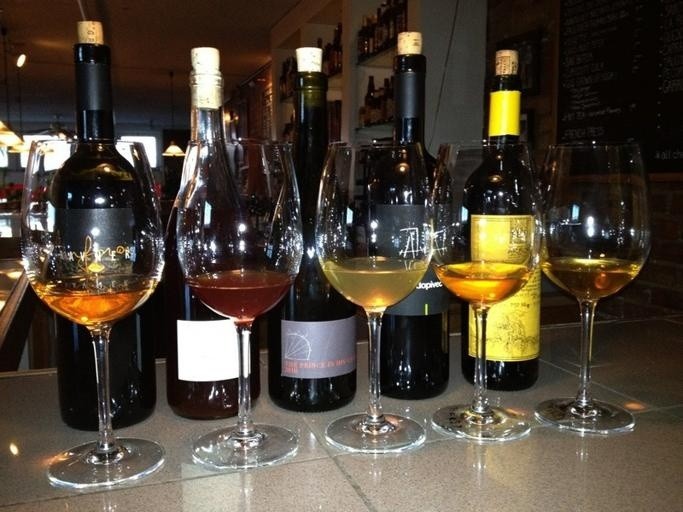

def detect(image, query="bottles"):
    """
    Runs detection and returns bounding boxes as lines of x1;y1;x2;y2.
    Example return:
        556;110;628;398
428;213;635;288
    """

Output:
356;1;406;62
49;18;158;429
278;22;342;100
362;73;401;125
464;48;541;391
270;46;358;413
379;31;449;400
162;45;261;421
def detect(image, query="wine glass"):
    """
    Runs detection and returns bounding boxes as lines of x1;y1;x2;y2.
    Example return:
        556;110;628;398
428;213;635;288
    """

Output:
174;141;300;474
20;139;167;494
535;139;652;435
316;137;427;455
430;146;542;444
